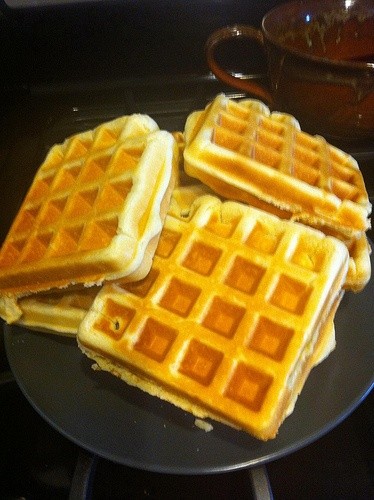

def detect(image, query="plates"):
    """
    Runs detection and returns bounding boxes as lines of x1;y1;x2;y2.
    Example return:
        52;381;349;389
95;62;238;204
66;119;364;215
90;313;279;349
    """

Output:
3;236;374;476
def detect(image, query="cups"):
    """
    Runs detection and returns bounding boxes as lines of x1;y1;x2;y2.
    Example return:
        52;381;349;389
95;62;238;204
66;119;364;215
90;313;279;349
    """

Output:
204;0;374;146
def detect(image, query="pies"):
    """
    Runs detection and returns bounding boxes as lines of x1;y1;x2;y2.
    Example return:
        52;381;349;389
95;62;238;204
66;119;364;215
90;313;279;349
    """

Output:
1;94;372;442
